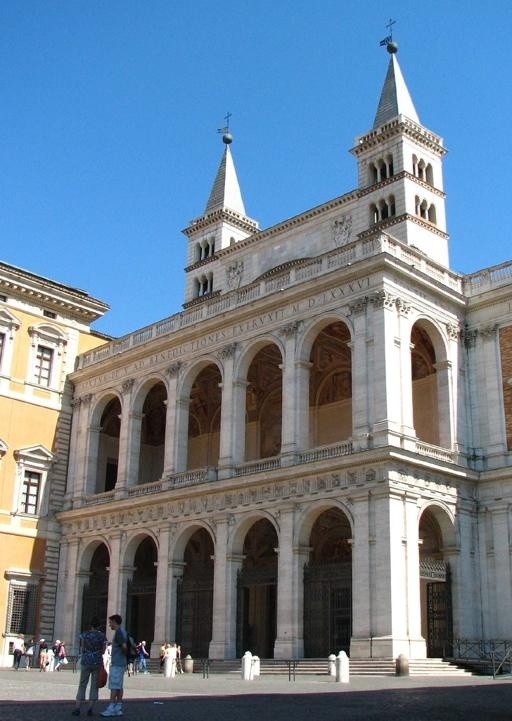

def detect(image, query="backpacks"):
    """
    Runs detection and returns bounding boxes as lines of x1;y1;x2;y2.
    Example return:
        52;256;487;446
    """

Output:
96;664;107;688
126;632;137;664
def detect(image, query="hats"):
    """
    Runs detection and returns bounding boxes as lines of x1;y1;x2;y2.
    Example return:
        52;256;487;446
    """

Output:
40;638;61;644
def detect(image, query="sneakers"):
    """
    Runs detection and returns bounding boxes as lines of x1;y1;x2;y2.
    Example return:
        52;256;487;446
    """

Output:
72;708;97;717
99;707;123;717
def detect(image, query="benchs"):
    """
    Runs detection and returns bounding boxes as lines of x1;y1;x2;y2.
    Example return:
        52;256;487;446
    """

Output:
58;656;80;674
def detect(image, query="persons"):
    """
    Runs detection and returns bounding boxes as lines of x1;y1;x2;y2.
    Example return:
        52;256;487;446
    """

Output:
12;614;185;717
